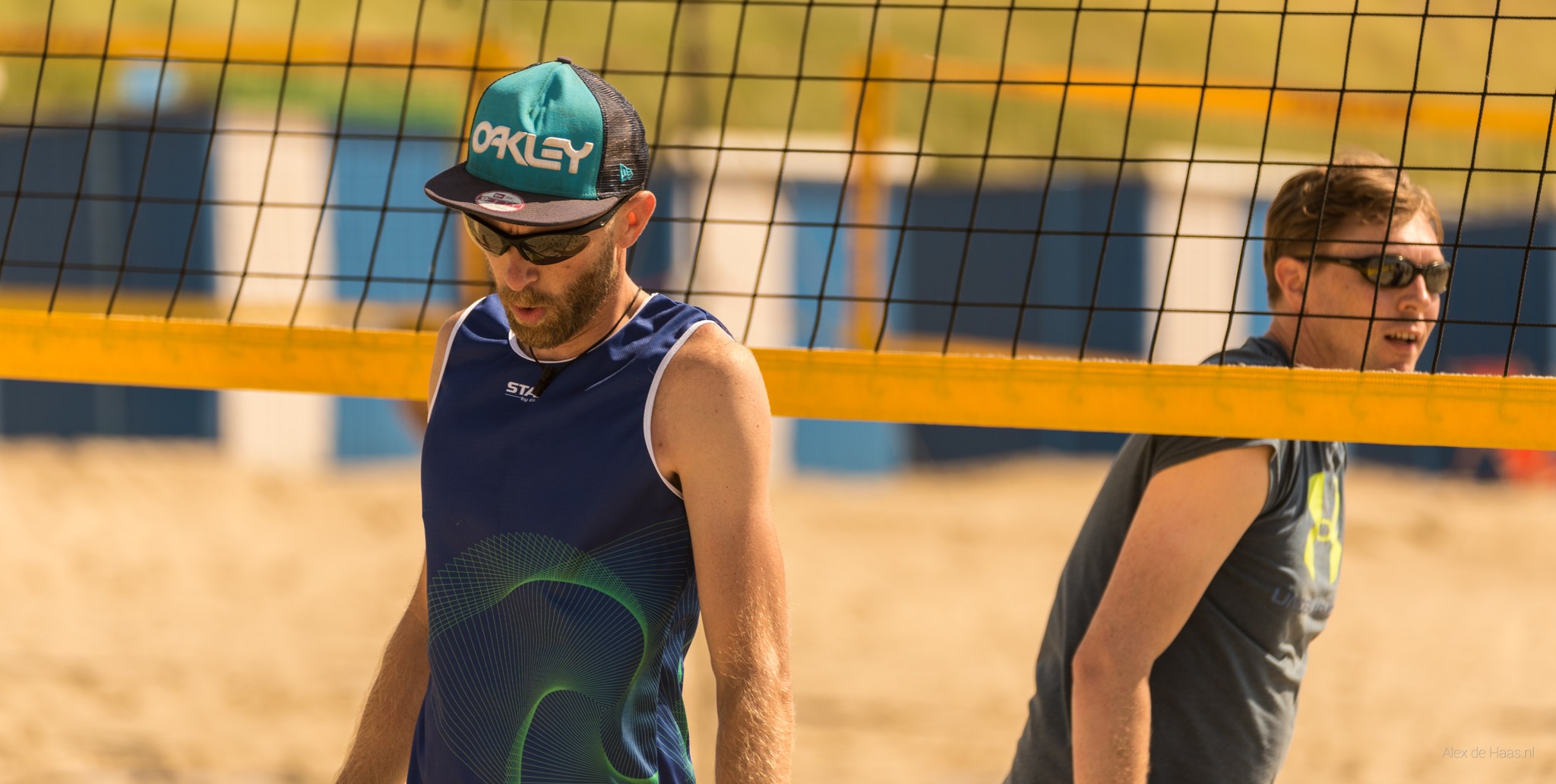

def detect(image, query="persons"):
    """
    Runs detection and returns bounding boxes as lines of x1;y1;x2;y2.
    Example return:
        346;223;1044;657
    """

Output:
339;56;794;784
1002;150;1452;784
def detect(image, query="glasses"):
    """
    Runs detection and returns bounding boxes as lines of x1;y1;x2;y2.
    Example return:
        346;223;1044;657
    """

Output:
460;197;629;266
1295;253;1450;295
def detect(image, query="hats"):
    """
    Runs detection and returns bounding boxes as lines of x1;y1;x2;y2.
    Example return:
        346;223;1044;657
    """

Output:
425;57;649;227
